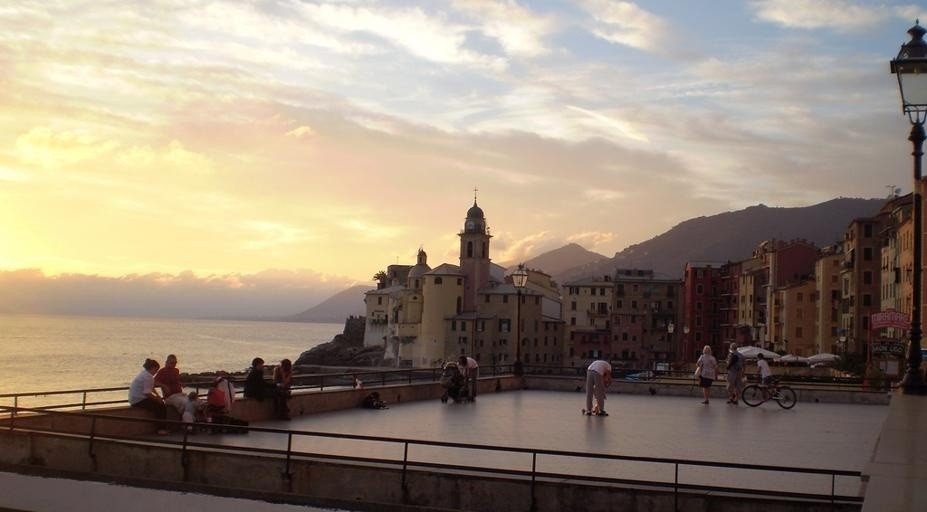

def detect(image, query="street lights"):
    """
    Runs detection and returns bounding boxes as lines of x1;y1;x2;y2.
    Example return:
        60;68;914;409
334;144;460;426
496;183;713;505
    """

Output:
510;262;528;375
890;19;925;396
664;321;690;376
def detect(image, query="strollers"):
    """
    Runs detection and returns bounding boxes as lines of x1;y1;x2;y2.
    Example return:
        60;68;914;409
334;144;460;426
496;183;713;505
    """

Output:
439;360;470;404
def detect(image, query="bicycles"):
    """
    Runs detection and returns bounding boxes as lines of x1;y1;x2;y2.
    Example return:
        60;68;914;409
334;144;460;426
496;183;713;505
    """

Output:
742;373;796;409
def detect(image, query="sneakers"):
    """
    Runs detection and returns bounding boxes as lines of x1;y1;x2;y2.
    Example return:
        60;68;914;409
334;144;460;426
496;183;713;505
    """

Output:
701;399;738;405
587;410;609;416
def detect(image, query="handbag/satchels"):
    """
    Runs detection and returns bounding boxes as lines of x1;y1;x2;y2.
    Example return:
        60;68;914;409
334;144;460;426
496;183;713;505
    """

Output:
695;367;702;376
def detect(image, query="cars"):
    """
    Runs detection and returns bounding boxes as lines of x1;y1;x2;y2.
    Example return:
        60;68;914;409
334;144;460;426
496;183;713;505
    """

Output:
610;359;672;379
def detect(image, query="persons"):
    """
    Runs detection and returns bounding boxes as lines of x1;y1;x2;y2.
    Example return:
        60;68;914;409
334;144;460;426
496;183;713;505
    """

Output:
586;359;613;417
154;354;202;414
694;345;720;405
273;359;292;397
456;355;480;401
724;342;746;405
244;357;292;421
755;353;773;400
593;377;610;416
179;391;203;431
203;370;235;433
128;358;173;436
